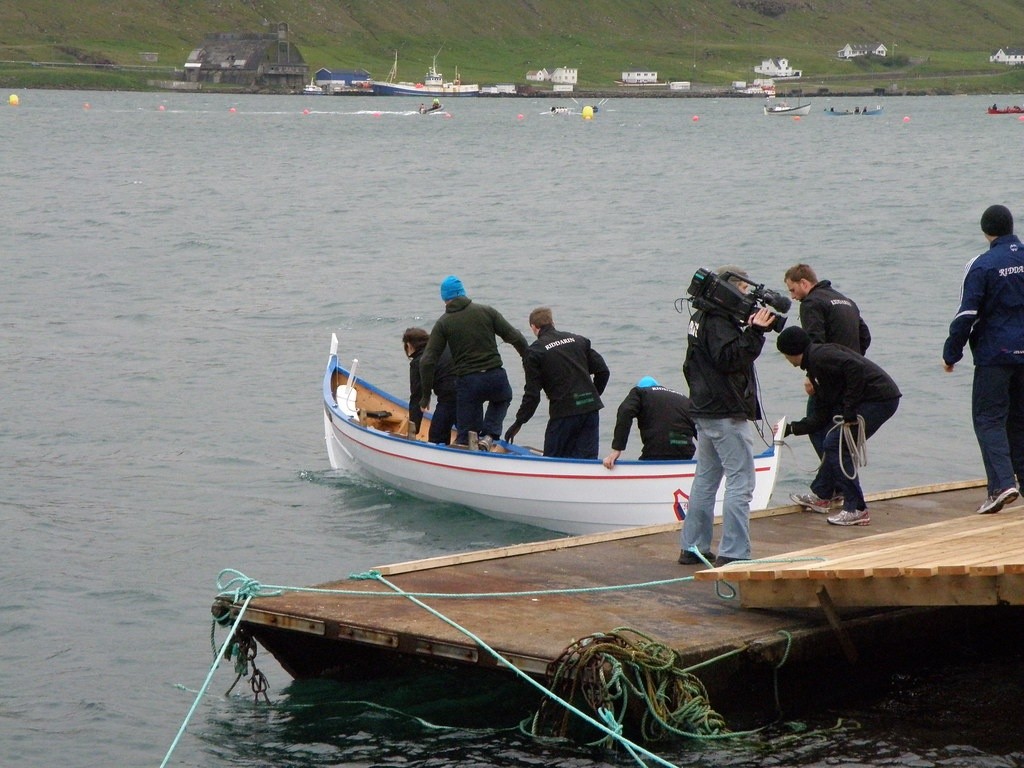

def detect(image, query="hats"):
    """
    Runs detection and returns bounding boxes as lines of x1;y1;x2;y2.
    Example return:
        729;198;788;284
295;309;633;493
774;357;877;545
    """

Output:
440;275;467;301
776;325;811;356
981;204;1014;236
638;376;658;388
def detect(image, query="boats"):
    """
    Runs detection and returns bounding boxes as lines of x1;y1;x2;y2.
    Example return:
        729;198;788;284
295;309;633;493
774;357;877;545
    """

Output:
824;108;854;115
303;77;330;95
368;49;479;97
550;107;567;114
419;103;444;114
856;108;885;116
764;103;811;116
321;332;788;538
592;108;598;112
730;78;776;98
988;107;1024;113
333;82;375;97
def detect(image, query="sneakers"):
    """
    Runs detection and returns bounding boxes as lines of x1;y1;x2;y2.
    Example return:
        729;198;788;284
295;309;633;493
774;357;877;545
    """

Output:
789;491;831;514
826;507;871;526
976;486;1019;514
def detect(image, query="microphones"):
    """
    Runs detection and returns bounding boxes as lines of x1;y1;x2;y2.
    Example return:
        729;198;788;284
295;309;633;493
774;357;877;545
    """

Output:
762;289;792;315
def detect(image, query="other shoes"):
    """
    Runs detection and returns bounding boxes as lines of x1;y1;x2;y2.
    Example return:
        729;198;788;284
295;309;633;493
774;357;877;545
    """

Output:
477;440;490;453
716;555;751;565
678;547;717;564
832;490;844;501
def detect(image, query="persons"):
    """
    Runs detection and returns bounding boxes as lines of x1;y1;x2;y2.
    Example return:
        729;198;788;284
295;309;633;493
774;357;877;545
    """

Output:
505;307;610;460
403;327;459;445
784;264;871;506
678;266;776;569
830;106;868;112
418;275;529;454
943;205;1024;514
603;376;698;470
774;326;903;526
992;103;997;110
